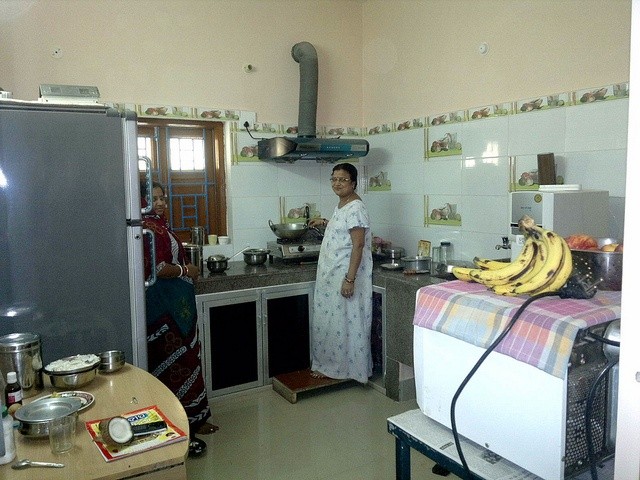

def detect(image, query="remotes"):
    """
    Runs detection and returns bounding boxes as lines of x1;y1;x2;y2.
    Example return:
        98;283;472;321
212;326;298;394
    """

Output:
132;421;168;437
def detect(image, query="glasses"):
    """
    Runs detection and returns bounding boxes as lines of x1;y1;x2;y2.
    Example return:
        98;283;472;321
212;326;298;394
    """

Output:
329;175;352;183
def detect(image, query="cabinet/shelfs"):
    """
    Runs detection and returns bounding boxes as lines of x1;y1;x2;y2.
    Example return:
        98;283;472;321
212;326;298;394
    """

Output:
194;281;315;404
366;284;387;395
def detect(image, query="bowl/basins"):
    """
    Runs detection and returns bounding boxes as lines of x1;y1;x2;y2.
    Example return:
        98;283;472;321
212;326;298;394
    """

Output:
99;360;125;374
218;237;229;245
208;235;217;246
570;237;622;292
386;247;404;259
43;355;100;390
98;350;125;362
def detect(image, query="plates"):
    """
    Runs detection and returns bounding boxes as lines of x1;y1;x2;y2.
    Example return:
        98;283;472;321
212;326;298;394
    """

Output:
32;390;94;411
380;263;403;271
12;397;82;421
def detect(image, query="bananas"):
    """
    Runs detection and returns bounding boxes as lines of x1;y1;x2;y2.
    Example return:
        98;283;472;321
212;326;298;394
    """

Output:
452;214;573;298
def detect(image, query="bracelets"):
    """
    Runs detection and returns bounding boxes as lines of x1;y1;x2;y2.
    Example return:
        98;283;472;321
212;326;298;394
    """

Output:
323;218;326;226
345;276;356;283
176;264;189;279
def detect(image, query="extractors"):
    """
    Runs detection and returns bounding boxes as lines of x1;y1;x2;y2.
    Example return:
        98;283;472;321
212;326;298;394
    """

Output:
257;42;370;161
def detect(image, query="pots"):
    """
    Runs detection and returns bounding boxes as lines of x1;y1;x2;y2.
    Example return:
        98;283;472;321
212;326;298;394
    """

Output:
269;220;324;238
15;412;78;439
401;254;430;275
243;249;269;266
206;255;229;274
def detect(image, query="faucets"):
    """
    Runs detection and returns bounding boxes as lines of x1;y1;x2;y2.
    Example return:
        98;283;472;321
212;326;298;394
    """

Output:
495;243;510;250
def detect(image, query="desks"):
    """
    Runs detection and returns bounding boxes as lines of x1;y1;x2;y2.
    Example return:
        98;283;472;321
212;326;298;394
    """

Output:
385;408;614;480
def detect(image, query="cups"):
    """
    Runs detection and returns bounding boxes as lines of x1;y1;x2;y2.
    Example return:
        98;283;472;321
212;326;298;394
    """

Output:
46;416;76;455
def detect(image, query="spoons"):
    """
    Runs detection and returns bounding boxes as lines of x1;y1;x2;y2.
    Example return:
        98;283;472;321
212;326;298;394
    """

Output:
11;460;64;471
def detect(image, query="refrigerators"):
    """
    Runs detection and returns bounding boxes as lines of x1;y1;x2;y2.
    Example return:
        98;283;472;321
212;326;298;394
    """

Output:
0;100;158;370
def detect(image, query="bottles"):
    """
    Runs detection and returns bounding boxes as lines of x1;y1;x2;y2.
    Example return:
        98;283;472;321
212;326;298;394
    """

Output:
441;242;452;265
5;373;21;407
1;407;16;465
432;247;440;274
184;246;203;276
191;226;204;244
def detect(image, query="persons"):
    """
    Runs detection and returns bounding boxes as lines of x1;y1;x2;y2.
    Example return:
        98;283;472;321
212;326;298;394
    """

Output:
309;162;374;384
138;180;219;435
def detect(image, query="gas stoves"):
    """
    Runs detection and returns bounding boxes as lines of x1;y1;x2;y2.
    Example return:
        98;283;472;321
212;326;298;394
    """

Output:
267;238;323;266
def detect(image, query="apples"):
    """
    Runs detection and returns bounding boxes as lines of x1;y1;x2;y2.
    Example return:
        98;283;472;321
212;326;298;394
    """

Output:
601;243;619;252
567;234;598;250
